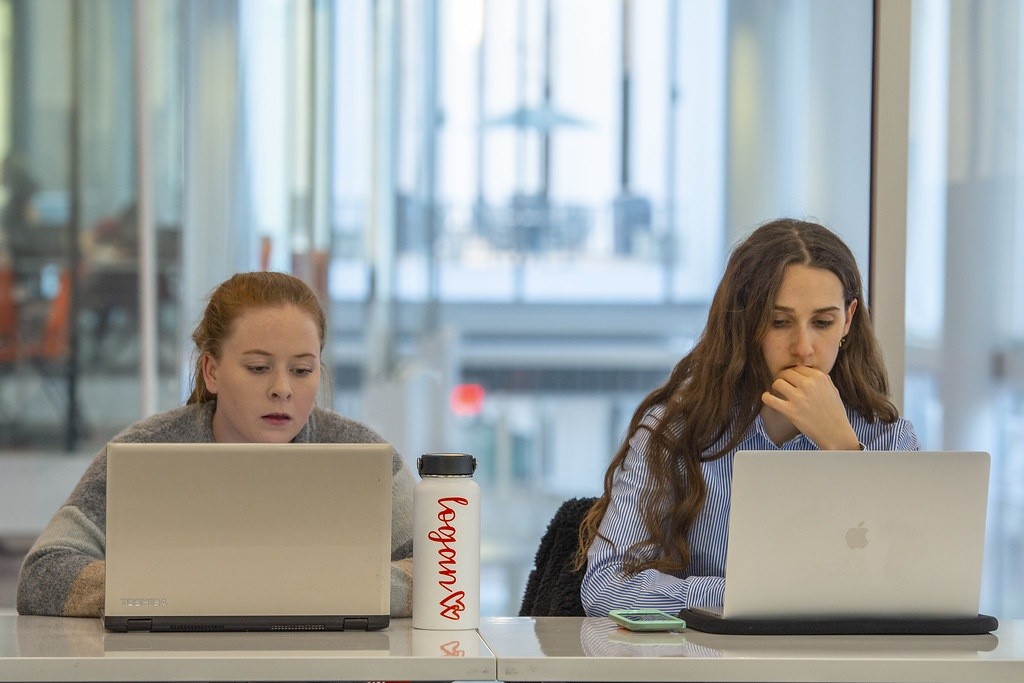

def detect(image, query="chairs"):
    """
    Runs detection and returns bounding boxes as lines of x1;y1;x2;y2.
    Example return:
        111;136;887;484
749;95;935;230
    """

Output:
0;219;330;456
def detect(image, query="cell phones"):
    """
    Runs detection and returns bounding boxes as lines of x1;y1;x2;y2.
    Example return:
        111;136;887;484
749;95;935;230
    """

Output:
609;609;687;632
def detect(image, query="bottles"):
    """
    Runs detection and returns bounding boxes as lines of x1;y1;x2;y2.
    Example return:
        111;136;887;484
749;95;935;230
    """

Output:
413;453;481;631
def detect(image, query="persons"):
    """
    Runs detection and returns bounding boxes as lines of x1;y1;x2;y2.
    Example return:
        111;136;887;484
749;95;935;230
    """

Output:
17;272;418;617
579;220;922;615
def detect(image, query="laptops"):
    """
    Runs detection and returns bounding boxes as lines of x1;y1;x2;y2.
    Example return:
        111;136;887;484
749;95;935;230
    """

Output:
97;442;394;634
693;450;992;621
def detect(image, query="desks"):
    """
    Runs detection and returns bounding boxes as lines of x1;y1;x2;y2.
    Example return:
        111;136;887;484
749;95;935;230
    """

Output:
1;605;498;683
91;263;175;360
475;614;1023;682
